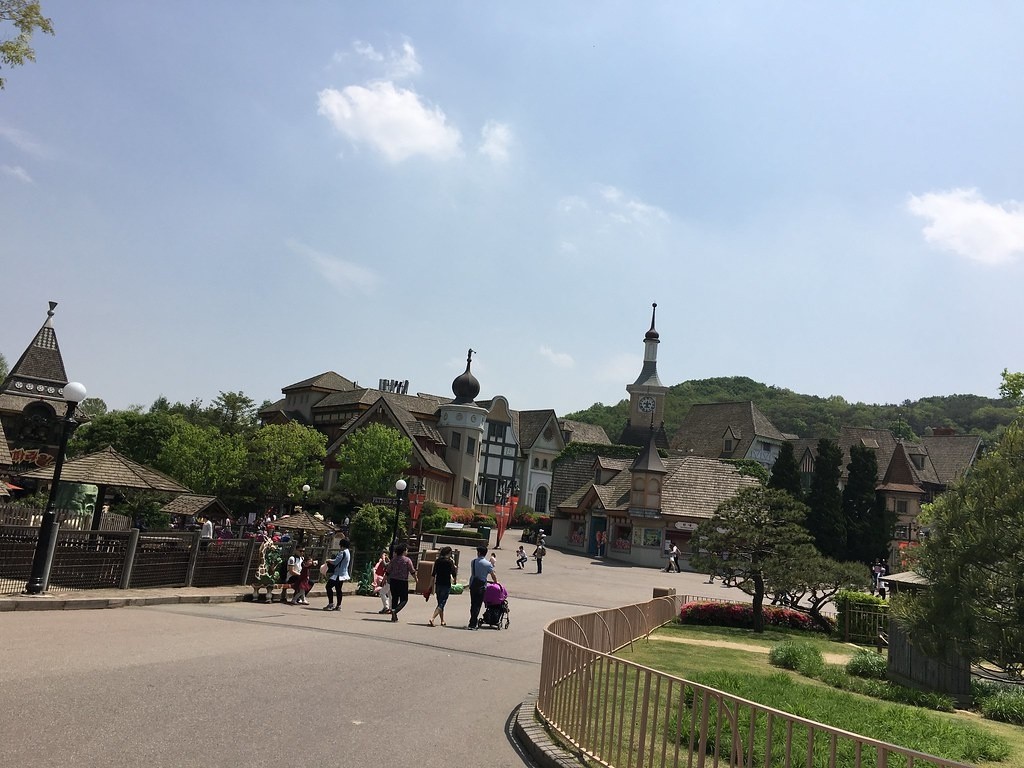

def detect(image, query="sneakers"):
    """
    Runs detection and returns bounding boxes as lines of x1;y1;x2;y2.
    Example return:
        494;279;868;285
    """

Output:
323;605;333;611
379;609;391;613
334;606;342;610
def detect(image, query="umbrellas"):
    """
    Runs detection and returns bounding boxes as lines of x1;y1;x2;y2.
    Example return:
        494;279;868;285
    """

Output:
267;510;341;545
12;443;195;552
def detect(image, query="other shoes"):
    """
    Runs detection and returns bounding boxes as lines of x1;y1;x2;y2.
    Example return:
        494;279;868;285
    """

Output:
392;609;398;620
302;601;309;604
291;600;299;604
468;624;479;630
430;618;435;626
441;622;446;625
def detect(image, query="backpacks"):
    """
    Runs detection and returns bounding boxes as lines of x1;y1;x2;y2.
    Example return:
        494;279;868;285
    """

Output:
542;547;546;556
677;549;681;556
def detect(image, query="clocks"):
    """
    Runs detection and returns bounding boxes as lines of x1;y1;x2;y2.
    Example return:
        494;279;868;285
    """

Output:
639;396;655;412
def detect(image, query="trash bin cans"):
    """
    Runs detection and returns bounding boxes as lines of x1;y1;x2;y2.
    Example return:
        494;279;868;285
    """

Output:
415;561;435;595
477;526;491;542
653;587;676;599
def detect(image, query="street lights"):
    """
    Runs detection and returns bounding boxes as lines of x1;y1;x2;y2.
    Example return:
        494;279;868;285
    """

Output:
388;479;407;559
296;484;311;549
23;382;87;594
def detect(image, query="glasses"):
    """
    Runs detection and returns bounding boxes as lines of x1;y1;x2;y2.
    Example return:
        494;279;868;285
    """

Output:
297;550;306;553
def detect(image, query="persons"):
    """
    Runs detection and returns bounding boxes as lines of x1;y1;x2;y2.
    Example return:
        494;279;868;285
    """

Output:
515;546;528;569
536;540;546;572
871;557;890;601
286;547;318;603
427;547;457;626
378;545;419;622
467;546;496;630
322;538;351;611
200;507;349;550
659;541;681;573
521;528;546;546
291;557;318;606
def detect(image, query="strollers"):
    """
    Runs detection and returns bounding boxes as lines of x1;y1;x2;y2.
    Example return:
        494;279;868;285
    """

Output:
477;580;511;630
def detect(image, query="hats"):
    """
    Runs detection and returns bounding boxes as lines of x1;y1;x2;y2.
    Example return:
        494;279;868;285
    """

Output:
424;587;432;602
320;561;327;577
542;534;546;537
539;529;544;532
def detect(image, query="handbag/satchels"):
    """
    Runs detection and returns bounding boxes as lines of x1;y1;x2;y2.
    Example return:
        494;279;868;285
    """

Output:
469;577;485;592
484;608;499;624
326;554;336;573
670;557;674;562
